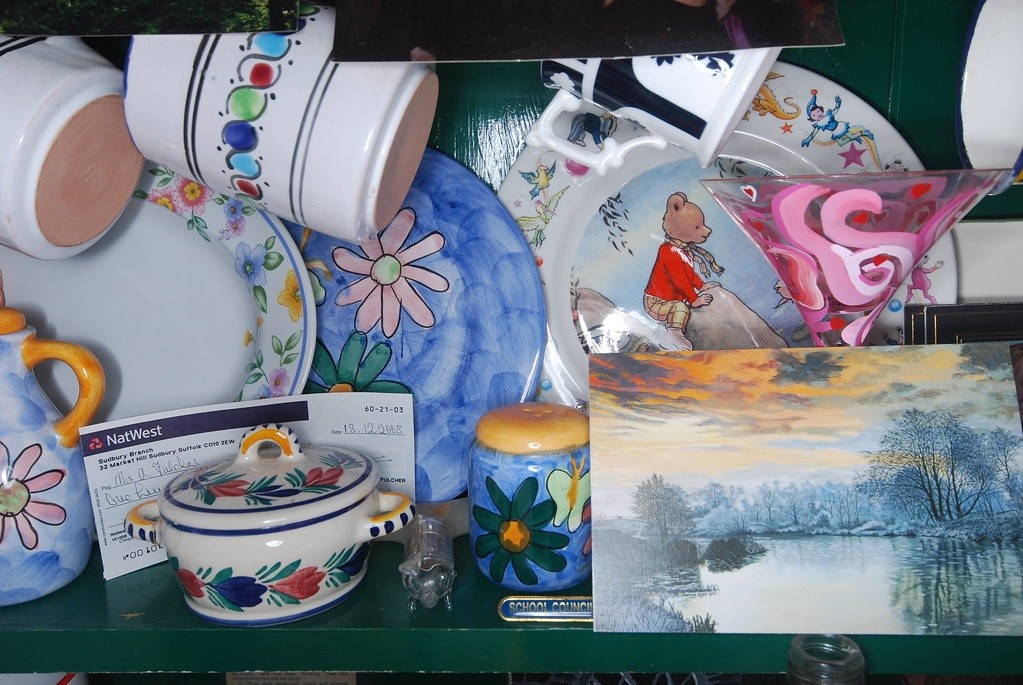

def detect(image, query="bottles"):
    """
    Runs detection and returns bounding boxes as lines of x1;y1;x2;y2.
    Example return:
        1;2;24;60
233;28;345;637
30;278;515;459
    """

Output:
466;405;593;595
0;307;108;608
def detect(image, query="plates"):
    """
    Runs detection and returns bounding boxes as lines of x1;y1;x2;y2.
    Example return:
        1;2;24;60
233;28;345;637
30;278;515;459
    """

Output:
0;59;962;504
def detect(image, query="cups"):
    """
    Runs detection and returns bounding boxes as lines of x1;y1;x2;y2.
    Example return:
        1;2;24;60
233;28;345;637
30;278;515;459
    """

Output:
0;35;147;261
125;1;442;243
541;50;781;168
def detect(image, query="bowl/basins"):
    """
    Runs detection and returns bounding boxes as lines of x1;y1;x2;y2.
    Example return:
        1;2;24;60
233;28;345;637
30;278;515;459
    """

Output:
126;424;421;626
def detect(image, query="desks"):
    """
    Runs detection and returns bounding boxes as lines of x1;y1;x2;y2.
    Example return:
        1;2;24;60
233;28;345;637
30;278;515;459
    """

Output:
0;534;1023;684
